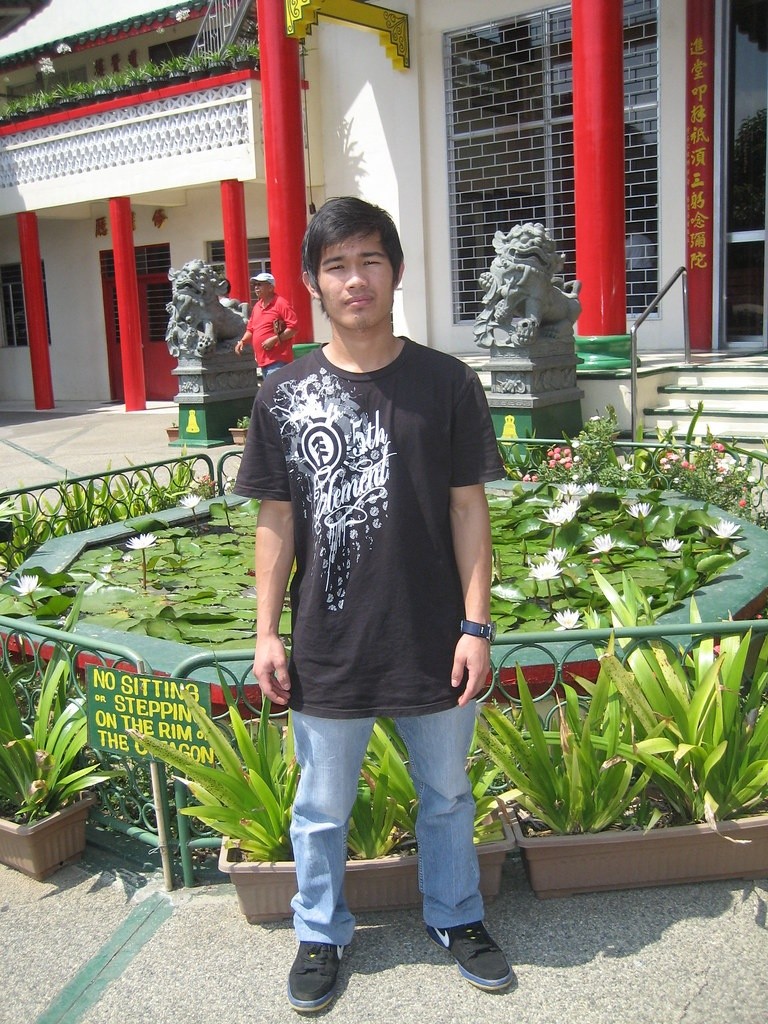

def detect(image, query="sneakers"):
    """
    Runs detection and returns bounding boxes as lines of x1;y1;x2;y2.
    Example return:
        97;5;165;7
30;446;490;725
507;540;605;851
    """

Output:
425;921;513;991
285;940;345;1012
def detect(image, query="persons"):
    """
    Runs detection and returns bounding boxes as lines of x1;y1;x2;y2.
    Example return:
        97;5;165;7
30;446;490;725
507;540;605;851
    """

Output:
233;272;300;380
231;198;517;1014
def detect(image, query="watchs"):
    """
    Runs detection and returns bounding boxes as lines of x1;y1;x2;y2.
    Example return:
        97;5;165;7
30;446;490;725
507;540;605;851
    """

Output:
457;618;499;645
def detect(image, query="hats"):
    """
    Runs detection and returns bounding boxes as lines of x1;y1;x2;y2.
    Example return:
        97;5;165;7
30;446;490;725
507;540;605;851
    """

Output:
250;273;276;288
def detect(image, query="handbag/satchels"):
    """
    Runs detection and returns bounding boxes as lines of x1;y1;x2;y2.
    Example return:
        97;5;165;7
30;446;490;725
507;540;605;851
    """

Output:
273;319;286;346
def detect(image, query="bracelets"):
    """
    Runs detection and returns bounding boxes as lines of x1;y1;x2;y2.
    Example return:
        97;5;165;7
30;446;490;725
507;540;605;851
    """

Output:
240;339;247;347
277;334;281;343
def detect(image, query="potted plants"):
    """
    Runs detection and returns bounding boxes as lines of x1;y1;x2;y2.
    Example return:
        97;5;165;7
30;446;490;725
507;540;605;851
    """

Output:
222;40;261;71
142;60;172;90
228;416;250;445
134;667;526;927
0;582;129;882
164;423;179;441
0;63;152;127
201;48;234;77
161;55;191;86
474;564;768;899
187;53;210;81
